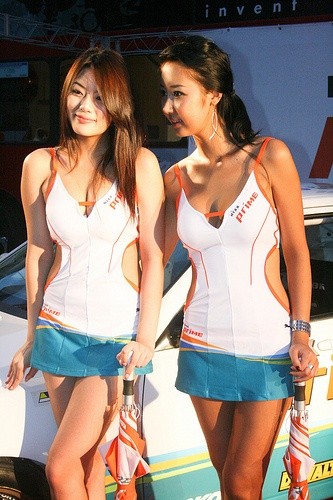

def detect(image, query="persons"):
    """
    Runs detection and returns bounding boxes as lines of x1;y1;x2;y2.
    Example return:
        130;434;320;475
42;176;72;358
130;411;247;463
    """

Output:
5;48;166;500
156;35;319;500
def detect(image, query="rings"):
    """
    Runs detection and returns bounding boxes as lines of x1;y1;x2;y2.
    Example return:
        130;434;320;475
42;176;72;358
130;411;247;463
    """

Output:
129;341;136;346
306;364;314;371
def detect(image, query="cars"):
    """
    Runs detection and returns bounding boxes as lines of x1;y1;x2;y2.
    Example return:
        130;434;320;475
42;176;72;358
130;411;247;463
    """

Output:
1;183;333;500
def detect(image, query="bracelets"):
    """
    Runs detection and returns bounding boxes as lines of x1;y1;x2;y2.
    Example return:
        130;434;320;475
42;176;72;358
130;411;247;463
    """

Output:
291;320;311;338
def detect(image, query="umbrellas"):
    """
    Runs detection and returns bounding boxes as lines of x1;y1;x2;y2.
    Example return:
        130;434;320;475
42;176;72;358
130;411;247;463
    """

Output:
283;357;316;500
98;349;152;500
